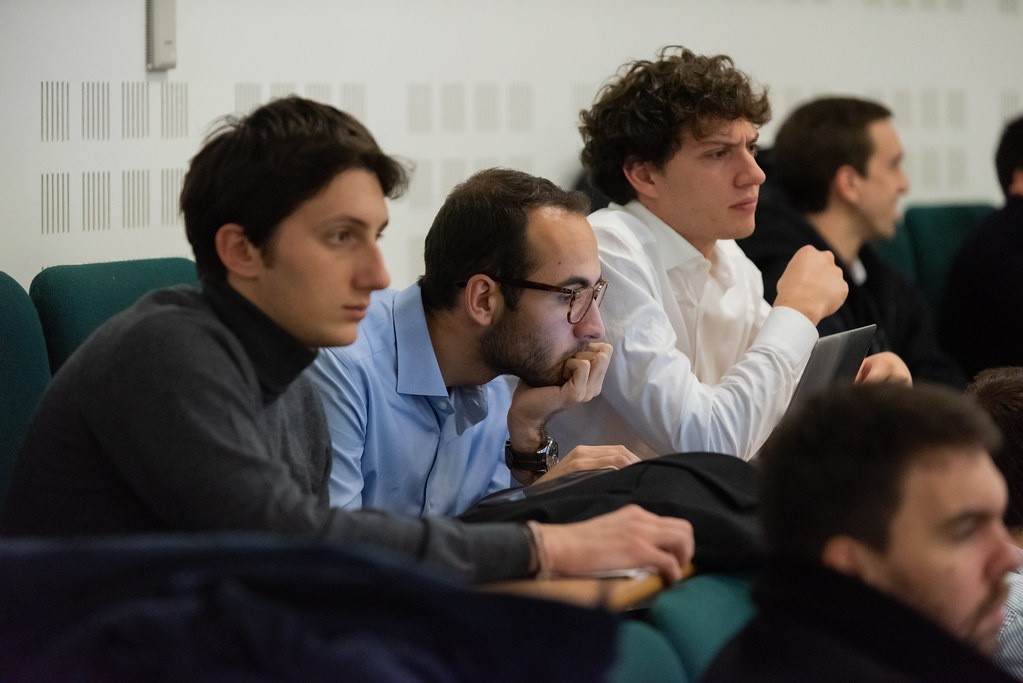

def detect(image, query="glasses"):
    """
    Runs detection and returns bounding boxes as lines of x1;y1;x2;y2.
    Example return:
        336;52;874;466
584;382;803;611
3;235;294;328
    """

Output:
455;276;610;326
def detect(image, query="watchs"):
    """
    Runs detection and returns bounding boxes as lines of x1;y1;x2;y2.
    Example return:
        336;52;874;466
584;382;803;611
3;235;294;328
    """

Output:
505;434;558;474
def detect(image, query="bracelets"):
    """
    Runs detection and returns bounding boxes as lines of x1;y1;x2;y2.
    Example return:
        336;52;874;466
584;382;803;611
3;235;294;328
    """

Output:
525;520;549;582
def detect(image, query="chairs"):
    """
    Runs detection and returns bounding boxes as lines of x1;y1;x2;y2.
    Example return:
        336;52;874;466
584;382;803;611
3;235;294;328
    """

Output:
0;270;53;503
871;202;1001;303
595;568;776;683
28;257;202;381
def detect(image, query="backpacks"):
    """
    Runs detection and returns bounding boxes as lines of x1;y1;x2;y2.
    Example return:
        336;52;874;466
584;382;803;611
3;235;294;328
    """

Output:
462;448;764;606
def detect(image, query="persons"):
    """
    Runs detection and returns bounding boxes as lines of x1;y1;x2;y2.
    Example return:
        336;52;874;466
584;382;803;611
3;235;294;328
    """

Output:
543;45;911;464
935;114;1023;367
305;166;642;521
734;96;970;392
691;382;1023;683
967;368;1023;683
1;96;698;587
